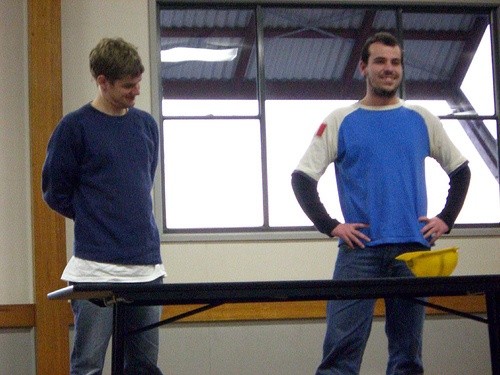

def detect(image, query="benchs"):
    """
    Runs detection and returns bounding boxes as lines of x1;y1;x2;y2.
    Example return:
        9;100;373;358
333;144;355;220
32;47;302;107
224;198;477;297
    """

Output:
47;274;500;375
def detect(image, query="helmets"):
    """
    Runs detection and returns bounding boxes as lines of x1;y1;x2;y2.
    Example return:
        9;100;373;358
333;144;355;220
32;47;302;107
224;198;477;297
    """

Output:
395;246;459;277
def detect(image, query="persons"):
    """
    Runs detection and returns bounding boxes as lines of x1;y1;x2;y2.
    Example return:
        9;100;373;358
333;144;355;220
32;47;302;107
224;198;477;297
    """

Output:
291;31;471;375
41;37;168;375
